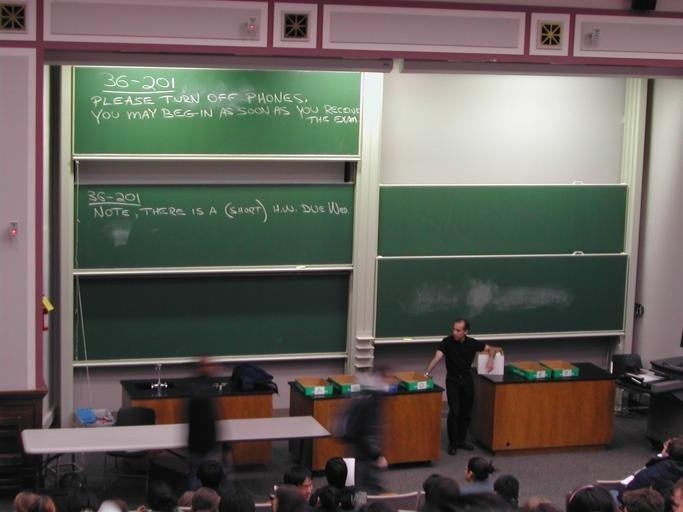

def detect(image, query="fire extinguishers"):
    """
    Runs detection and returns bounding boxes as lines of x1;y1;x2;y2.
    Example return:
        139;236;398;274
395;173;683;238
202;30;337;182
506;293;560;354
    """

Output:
41;295;53;330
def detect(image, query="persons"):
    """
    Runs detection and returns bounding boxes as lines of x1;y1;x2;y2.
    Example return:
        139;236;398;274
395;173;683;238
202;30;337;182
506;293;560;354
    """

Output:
12;442;394;510
422;316;504;457
420;438;683;512
184;356;225;493
348;367;392;493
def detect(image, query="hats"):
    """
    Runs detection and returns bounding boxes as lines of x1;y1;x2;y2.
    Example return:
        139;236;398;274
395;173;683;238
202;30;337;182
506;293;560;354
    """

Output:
192;487;222;512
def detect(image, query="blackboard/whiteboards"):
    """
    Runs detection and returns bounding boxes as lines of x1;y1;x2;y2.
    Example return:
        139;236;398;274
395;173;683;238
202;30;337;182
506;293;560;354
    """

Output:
72;272;352;365
74;184;356;270
72;67;363;160
373;257;630;341
379;184;630;255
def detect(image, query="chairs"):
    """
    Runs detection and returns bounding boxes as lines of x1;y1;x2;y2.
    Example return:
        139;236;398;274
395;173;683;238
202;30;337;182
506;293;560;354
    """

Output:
611;353;651;416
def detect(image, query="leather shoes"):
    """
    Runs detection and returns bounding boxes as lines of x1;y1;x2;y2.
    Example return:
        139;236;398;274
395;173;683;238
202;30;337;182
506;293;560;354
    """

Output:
455;441;473;450
448;443;457;455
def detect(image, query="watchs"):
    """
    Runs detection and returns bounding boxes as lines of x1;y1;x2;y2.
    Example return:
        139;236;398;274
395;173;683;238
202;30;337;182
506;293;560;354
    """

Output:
424;369;430;377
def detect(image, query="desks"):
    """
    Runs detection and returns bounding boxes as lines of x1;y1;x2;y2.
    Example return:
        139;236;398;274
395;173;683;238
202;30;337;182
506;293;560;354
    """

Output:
21;414;331;487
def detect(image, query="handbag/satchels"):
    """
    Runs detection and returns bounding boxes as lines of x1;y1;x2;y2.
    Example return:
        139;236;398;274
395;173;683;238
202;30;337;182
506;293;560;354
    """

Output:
232;365;279;393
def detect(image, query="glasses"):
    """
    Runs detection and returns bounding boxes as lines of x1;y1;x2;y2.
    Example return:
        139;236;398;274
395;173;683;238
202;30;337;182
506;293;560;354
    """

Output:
269;495;278;500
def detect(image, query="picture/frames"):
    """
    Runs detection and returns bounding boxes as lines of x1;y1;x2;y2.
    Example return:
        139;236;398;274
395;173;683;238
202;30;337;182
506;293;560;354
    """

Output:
1;2;28;34
279;10;311;41
537;19;564;50
